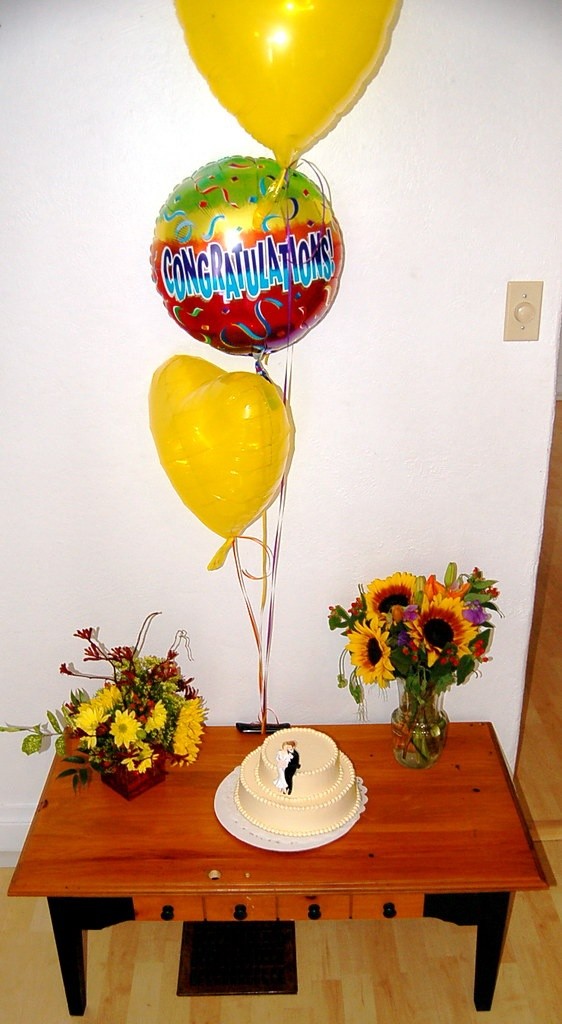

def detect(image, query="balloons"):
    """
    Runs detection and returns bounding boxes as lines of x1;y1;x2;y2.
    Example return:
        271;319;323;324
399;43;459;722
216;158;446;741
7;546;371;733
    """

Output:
173;1;399;226
149;155;344;376
148;355;292;570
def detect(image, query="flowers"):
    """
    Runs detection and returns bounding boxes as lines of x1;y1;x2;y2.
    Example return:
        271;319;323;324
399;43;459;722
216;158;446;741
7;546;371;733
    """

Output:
0;612;210;793
327;562;505;763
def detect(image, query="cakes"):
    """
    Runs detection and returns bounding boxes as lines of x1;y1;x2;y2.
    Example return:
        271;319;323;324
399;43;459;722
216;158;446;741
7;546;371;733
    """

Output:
234;727;362;836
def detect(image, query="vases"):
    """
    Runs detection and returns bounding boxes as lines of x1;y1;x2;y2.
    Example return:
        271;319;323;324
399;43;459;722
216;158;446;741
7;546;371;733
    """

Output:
390;677;453;769
101;743;167;801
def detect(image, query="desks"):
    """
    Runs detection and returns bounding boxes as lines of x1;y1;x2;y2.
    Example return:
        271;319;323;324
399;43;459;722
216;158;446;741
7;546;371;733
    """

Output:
7;722;550;1016
